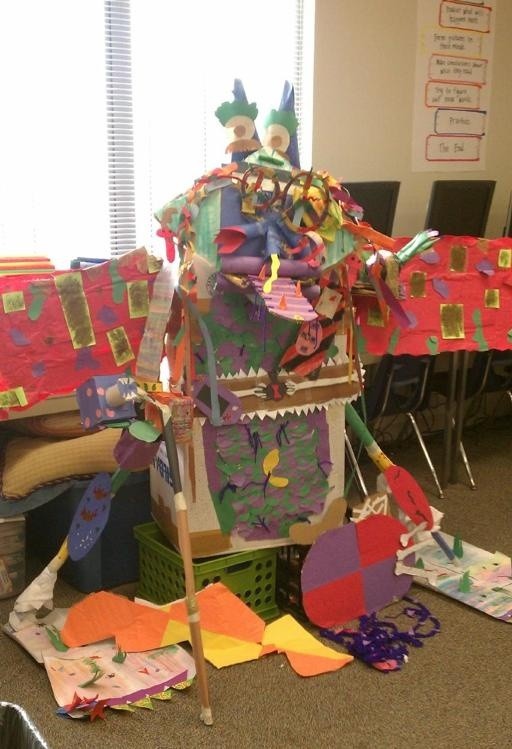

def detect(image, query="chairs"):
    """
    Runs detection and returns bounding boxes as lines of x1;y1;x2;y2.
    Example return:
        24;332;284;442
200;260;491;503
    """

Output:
336;350;512;505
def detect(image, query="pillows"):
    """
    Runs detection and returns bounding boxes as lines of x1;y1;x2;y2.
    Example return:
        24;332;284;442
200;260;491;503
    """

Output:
0;407;158;518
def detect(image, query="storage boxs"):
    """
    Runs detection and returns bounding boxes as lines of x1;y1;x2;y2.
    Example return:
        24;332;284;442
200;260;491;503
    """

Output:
0;514;27;599
142;171;362;561
29;472;151;594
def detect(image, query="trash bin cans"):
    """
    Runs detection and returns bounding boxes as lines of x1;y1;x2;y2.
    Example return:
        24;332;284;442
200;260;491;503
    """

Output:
0;701;47;748
